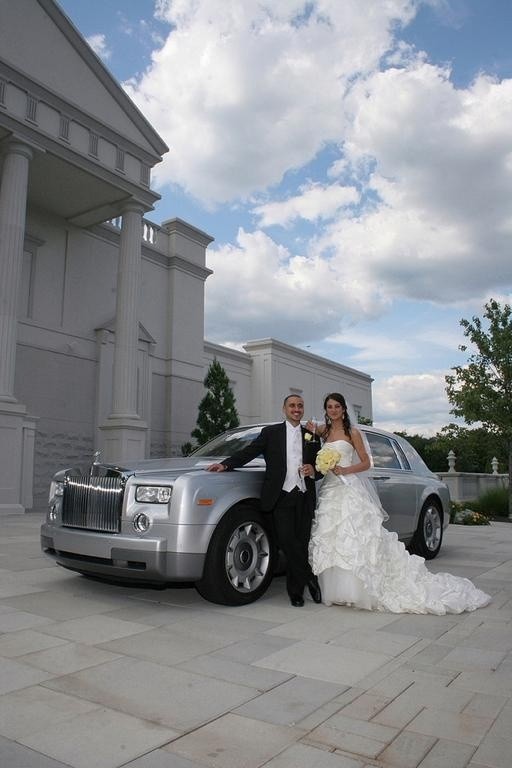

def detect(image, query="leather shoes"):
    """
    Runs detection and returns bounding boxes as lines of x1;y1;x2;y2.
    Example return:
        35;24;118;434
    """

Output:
291;595;304;608
308;575;322;603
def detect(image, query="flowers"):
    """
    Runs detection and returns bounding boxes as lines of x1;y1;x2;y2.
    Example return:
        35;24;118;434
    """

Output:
304;433;313;448
315;448;346;485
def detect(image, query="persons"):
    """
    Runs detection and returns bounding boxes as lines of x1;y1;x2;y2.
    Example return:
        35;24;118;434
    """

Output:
205;394;326;608
304;391;383;613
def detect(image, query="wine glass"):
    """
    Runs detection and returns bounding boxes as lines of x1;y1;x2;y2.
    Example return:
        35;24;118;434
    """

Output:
297;463;309;492
309;415;319;443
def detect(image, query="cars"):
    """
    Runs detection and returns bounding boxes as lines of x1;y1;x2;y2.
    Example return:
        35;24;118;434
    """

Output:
37;413;454;611
